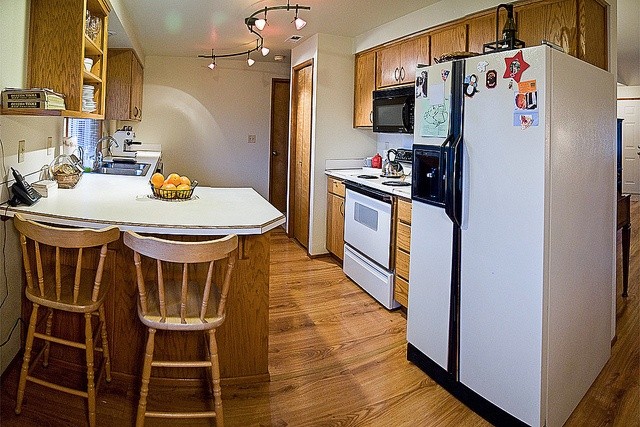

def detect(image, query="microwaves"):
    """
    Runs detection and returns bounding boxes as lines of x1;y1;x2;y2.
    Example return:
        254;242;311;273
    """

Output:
371;89;417;134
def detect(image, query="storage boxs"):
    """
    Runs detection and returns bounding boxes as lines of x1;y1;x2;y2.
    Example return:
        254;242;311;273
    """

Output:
395;201;412;310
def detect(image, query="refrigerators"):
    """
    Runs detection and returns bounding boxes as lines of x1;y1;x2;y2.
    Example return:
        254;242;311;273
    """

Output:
406;45;617;426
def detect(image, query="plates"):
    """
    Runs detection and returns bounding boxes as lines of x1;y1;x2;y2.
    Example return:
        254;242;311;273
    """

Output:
81;84;98;113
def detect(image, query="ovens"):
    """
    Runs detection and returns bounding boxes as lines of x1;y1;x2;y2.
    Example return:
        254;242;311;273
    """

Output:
341;179;397;270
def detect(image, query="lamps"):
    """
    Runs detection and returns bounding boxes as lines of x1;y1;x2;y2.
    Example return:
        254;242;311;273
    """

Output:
248;17;266;32
207;63;215;70
292;9;306;30
261;47;270;57
247;58;257;67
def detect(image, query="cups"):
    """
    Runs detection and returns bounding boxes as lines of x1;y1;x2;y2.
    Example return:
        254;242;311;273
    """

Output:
381;148;404;178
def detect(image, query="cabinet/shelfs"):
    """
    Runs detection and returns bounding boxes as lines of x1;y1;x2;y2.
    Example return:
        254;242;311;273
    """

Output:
352;46;376;131
430;15;469;69
105;47;144;121
404;268;410;271
0;1;116;121
327;172;345;262
375;27;430;89
468;7;496;52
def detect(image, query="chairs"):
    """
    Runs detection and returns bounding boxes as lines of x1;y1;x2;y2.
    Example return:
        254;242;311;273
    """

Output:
124;233;242;423
9;214;120;417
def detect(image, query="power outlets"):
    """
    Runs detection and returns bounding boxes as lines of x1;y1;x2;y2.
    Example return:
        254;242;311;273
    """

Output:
247;134;257;144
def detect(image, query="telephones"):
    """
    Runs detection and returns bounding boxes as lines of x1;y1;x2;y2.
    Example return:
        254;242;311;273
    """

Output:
10;167;41;206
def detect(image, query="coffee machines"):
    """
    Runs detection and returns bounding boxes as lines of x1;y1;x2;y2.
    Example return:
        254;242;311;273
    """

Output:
112;130;144;157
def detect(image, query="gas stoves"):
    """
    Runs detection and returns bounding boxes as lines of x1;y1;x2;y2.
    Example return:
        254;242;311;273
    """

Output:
348;148;415;199
